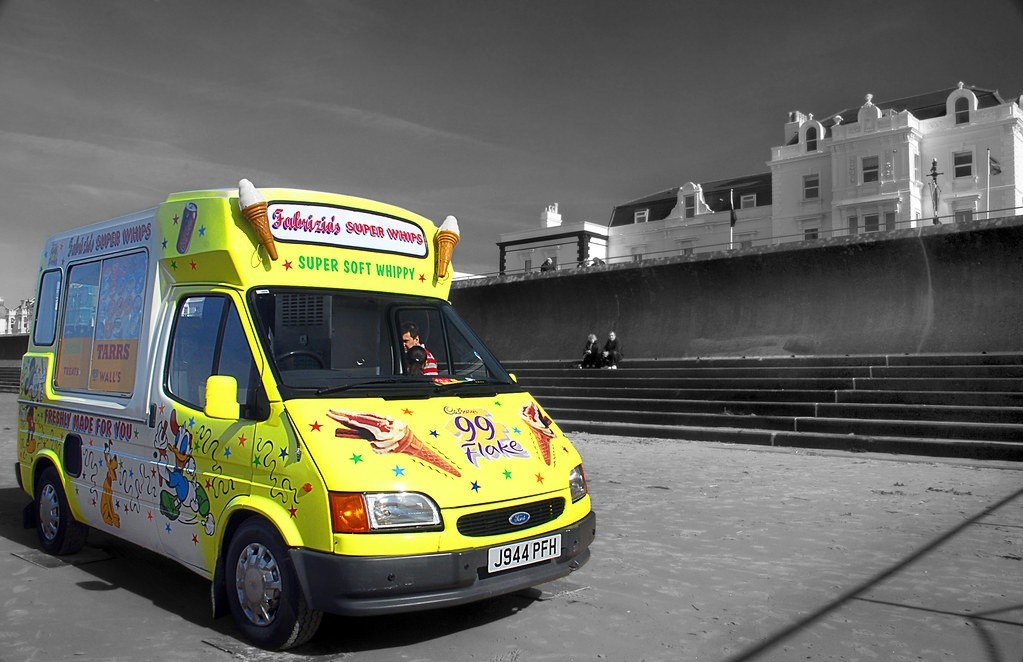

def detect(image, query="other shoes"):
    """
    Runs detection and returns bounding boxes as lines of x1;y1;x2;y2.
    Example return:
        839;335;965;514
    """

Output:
600;366;611;370
611;365;617;369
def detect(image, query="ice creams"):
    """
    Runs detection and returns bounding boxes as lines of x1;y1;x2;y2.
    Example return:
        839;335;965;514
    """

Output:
238;178;279;261
326;409;461;477
520;402;557;466
435;215;460;277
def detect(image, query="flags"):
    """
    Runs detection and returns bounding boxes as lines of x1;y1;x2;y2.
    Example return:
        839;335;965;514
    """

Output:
990;156;1002;176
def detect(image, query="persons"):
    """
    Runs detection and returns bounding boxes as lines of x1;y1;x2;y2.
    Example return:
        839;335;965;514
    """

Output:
400;321;438;376
541;258;556;272
601;330;624;369
583;333;599;368
591;257;605;265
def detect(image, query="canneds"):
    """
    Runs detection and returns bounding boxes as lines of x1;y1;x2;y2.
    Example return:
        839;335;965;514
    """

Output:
177;201;198;254
